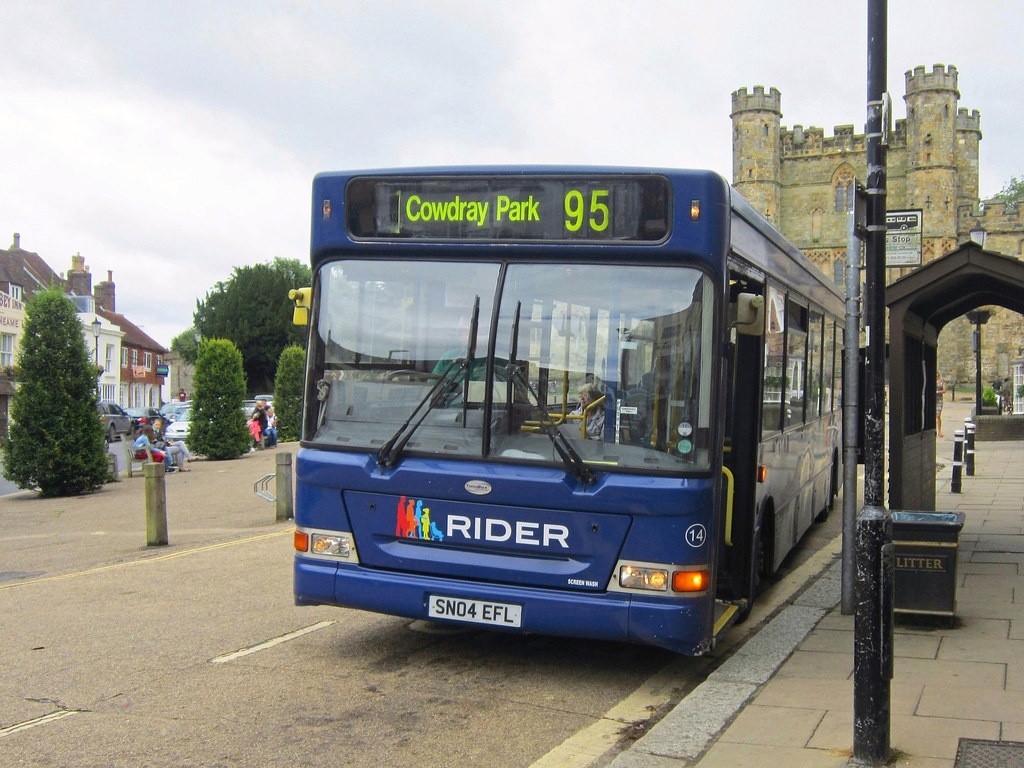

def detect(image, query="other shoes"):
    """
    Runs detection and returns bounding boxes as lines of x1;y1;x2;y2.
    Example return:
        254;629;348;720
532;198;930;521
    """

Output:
179;468;191;471
188;457;199;462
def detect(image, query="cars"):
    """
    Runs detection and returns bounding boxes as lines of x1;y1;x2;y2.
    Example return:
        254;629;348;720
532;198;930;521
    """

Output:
158;400;193;422
99;403;132;443
164;408;213;441
256;395;275;410
123;408;172;428
241;399;257;418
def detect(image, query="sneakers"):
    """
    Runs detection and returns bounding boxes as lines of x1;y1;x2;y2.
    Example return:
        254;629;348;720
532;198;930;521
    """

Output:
168;463;179;468
166;468;176;473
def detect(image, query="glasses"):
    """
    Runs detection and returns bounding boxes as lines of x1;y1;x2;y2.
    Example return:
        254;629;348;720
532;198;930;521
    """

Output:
579;394;588;398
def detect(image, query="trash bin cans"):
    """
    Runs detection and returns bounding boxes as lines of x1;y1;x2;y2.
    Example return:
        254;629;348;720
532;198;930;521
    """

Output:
887;509;966;628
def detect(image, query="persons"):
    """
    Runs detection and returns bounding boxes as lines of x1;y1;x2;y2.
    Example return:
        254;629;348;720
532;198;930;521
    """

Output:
132;418;199;472
992;376;1011;405
244;399;277;452
936;372;946;437
179;389;186;402
567;383;604;442
428;310;515;408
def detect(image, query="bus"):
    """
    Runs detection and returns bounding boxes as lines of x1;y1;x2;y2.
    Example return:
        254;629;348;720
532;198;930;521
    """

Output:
292;163;847;657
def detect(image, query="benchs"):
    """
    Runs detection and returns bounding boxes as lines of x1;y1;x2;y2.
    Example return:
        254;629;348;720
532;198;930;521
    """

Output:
120;433;178;478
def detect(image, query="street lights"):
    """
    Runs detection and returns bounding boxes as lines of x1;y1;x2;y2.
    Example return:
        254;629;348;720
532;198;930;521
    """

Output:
194;330;202;358
970;220;988;414
91;317;102;394
